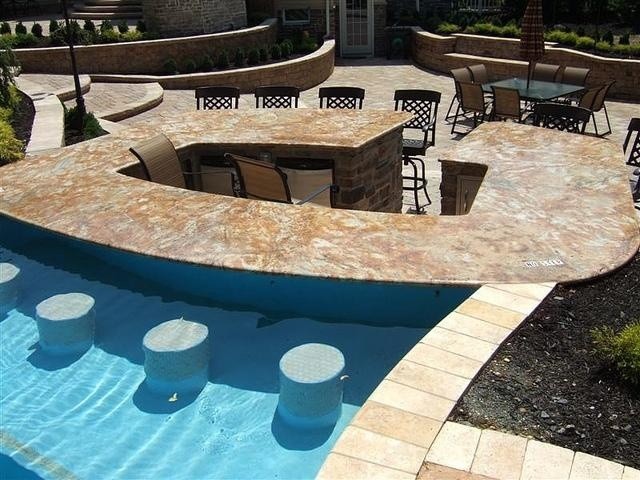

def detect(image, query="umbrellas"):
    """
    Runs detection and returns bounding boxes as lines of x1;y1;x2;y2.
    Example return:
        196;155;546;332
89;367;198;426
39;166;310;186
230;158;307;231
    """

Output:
519;0;547;88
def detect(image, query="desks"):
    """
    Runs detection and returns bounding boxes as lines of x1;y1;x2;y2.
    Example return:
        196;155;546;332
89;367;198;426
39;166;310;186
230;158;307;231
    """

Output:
482;78;586;102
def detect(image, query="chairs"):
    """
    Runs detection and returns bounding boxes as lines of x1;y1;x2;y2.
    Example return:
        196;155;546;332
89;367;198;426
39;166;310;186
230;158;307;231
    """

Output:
592;79;618;134
444;68;495;121
531;62;562;83
130;132;233;197
490;85;535;124
224;151;335;210
621;115;639;213
254;85;300;108
194;85;240;109
562;66;591;87
568;85;607;137
466;64;495;95
533;103;592;135
393;89;442;213
451;79;495;135
318;85;365;109
